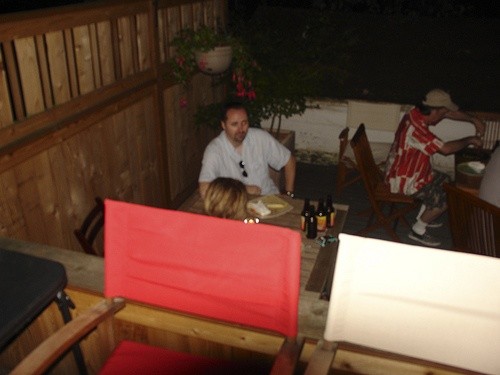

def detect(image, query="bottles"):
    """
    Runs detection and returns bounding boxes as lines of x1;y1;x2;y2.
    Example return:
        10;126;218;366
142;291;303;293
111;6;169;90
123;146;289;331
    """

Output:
305;205;318;239
315;199;328;232
301;196;310;232
325;197;336;228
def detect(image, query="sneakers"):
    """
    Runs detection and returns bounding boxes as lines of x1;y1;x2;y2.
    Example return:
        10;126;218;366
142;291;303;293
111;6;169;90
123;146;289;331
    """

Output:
408;226;440;246
424;221;443;227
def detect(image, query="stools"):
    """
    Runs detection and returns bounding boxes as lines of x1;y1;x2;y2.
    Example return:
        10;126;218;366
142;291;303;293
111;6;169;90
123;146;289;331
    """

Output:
0;248;87;375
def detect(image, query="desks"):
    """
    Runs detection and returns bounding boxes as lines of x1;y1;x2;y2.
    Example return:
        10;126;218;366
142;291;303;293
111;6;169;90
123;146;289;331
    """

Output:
177;188;349;294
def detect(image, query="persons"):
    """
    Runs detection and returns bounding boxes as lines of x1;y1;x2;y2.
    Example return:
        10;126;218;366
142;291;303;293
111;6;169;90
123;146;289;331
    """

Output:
384;90;486;246
198;103;296;200
203;177;247;220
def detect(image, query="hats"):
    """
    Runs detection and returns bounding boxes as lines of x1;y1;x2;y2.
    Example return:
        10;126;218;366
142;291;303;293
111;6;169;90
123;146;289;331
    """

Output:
422;89;459;112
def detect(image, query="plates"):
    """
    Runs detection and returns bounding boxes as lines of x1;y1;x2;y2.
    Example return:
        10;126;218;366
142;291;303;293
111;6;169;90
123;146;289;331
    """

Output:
246;194;293;219
457;161;487;177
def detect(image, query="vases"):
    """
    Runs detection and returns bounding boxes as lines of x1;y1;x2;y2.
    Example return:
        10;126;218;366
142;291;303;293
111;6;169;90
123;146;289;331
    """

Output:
195;46;233;73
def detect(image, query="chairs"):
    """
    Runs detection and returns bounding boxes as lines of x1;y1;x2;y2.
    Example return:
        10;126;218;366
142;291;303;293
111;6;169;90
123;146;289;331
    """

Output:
8;198;306;375
338;124;416;242
442;183;500;259
474;112;500;151
302;233;500;375
75;196;104;256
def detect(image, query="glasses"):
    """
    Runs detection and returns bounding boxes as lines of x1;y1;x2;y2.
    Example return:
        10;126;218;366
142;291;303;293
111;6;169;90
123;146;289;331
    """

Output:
240;160;247;177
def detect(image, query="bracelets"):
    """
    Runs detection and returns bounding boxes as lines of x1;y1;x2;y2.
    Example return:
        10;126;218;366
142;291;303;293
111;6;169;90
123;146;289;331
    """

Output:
283;191;294;198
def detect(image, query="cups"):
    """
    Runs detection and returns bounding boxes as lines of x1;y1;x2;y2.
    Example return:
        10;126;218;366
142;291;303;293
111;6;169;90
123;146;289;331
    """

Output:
244;216;260;224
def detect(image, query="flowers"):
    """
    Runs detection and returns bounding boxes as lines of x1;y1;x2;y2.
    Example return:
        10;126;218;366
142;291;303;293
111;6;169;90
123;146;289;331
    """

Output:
168;24;257;108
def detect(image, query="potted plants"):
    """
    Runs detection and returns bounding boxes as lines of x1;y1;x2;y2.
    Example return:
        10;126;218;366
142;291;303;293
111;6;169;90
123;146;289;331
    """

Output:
246;18;358;193
